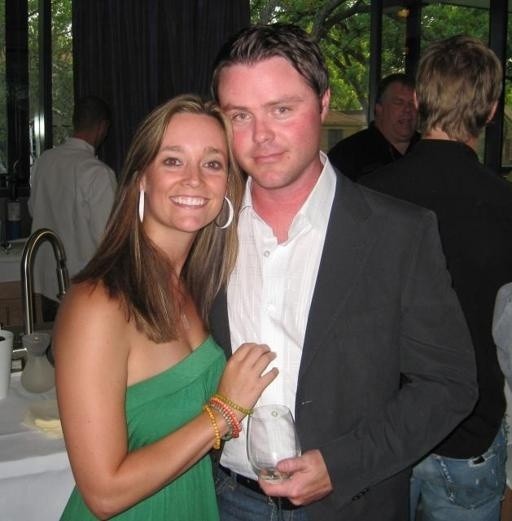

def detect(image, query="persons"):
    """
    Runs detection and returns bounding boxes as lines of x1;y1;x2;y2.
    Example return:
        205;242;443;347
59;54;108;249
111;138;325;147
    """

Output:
51;94;280;520
195;21;478;520
324;71;422;185
350;32;510;520
30;93;119;323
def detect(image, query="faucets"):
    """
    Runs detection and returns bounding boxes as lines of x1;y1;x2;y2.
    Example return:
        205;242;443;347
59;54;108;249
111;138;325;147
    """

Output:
19;226;69;336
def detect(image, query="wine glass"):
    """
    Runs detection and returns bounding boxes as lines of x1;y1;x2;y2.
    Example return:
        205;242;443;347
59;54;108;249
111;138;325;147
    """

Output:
245;402;303;520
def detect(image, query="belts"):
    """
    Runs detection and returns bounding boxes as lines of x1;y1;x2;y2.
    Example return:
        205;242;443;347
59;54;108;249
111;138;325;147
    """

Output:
217;460;273;499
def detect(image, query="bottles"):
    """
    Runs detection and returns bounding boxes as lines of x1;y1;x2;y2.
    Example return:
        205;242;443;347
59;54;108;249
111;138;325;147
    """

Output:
19;331;57;394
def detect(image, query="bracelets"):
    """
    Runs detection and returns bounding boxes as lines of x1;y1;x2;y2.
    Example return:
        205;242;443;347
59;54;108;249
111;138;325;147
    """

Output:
203;393;254;453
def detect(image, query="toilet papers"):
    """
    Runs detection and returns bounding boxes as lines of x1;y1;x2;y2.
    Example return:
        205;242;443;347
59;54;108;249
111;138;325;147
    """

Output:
0;326;13;401
